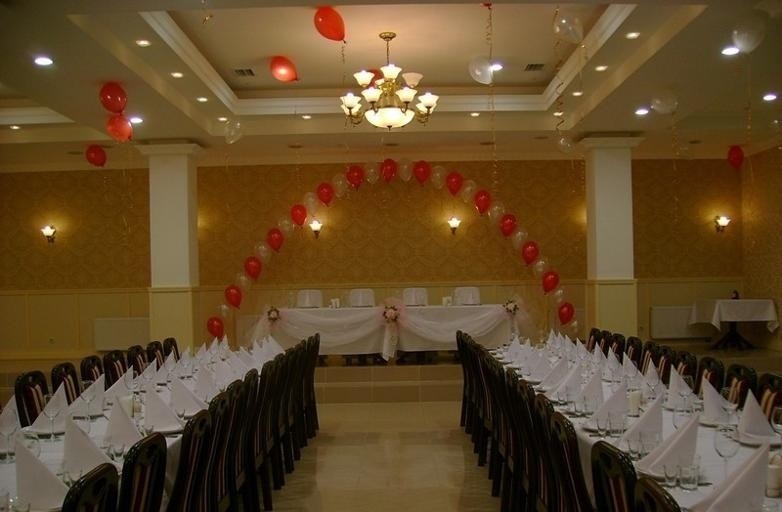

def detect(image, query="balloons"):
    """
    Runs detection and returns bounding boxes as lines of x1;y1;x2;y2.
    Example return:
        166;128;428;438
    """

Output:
244;217;295;280
475;189;529;252
107;114;132;143
344;159;414;187
98;83;127;114
732;13;765;53
553;10;583;45
468;56;493;85
652;93;677;113
521;242;582;337
314;5;346;43
85;144;106;166
414;161;478;205
728;145;744;169
271;55;297;83
290;175;346;228
207;272;251;342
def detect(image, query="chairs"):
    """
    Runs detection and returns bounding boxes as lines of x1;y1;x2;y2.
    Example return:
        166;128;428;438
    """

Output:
341;288;378;366
448;286;481;364
395;287;440;365
297;290;329;367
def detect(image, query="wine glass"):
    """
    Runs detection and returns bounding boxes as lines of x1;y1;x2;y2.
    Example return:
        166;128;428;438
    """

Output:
497;331;782;492
0;345;249;464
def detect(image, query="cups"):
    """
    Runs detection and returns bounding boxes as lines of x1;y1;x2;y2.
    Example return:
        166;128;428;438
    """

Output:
442;295;452;306
0;383;228;512
330;297;340;309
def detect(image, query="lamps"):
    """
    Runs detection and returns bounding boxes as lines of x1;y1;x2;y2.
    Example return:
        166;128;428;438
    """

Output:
340;32;439;132
308;220;323;239
40;224;56;243
715;216;731;232
447;217;461;235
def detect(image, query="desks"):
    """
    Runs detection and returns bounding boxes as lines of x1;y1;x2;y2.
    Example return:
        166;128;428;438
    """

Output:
687;298;780;351
250;304;519;364
